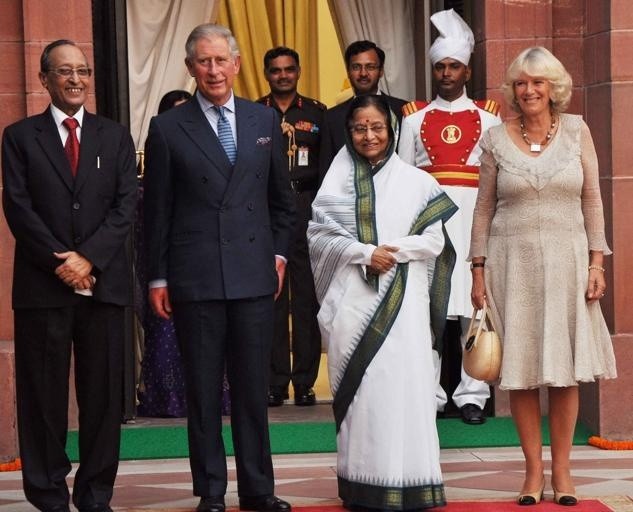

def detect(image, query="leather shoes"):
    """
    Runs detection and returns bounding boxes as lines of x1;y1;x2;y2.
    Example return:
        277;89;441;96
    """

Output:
240;495;290;512
266;390;289;406
295;386;316;405
462;404;485;424
551;475;577;505
197;496;224;512
517;475;545;505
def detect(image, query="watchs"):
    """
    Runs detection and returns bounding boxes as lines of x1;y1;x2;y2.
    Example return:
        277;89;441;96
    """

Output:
470;263;484;270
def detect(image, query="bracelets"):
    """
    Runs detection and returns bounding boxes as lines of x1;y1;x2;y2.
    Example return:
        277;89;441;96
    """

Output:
588;265;605;273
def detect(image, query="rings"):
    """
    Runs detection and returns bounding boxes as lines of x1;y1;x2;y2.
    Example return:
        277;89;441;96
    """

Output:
600;293;605;297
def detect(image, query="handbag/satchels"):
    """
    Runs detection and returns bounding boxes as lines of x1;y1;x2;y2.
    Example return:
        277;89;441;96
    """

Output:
463;300;502;381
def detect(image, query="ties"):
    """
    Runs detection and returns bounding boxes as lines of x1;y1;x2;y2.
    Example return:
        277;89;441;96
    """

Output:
63;118;80;177
212;106;237;165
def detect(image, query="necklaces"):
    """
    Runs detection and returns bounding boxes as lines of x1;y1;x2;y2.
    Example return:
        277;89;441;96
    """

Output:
520;110;555;152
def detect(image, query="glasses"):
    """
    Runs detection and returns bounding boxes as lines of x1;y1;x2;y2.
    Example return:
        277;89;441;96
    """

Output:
46;68;92;78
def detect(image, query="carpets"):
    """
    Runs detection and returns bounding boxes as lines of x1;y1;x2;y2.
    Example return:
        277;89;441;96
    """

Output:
58;415;596;463
220;497;616;512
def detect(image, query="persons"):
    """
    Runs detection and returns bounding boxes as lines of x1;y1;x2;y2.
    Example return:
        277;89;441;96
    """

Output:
465;45;613;506
141;24;300;512
131;88;232;418
2;38;140;512
397;10;504;425
306;94;462;512
311;41;409;202
251;47;328;406
